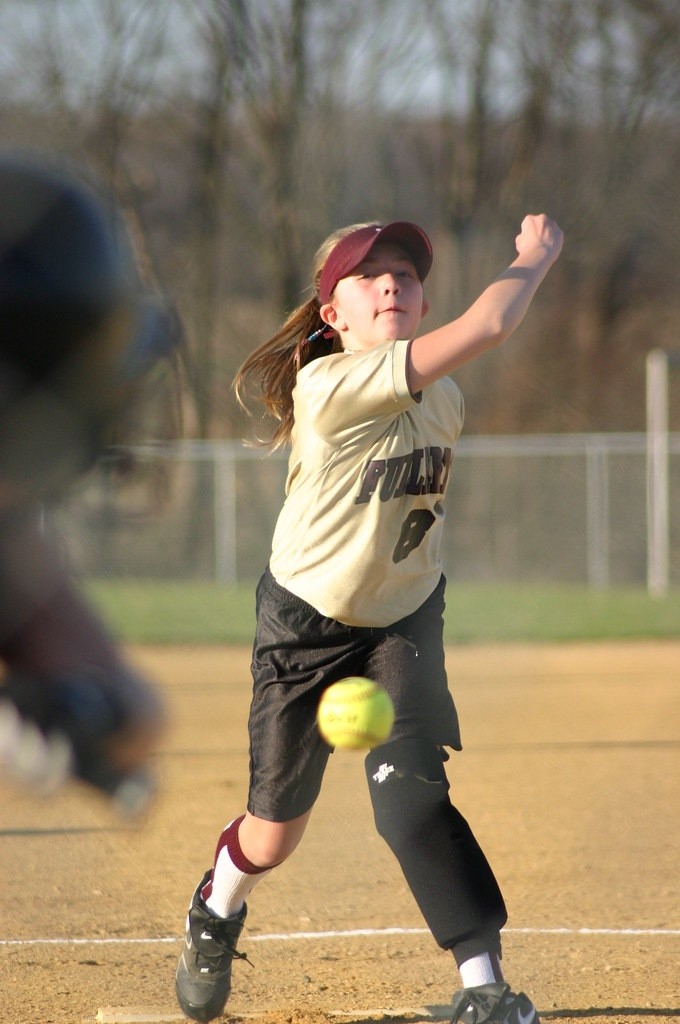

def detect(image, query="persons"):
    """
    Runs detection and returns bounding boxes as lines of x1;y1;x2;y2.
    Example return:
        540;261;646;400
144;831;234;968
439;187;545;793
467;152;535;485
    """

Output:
174;212;564;1022
0;162;174;817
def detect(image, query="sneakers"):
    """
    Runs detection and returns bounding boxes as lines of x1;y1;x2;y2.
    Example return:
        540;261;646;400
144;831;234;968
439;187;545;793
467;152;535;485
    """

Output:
449;983;540;1024
176;865;248;1022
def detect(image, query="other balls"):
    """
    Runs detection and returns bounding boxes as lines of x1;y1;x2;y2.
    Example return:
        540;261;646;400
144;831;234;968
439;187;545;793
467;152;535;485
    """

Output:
318;676;396;750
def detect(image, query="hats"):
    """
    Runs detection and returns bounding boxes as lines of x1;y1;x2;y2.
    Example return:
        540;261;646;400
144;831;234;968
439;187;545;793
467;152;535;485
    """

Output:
319;221;434;306
